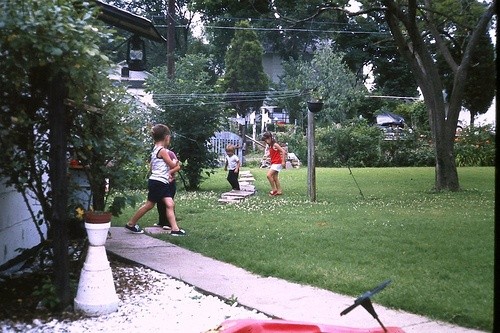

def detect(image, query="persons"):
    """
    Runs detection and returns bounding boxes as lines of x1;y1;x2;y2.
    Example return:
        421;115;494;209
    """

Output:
70;149;109;212
261;132;284;197
126;124;186;236
224;143;241;191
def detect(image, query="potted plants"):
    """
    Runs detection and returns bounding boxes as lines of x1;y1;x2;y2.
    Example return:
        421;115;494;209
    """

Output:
86;209;112;222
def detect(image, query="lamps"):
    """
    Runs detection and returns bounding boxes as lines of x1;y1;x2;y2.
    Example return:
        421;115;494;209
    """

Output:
127;31;146;71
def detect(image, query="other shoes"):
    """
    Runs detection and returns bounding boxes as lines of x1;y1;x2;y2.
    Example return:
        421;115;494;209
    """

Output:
163;225;171;229
154;223;162;226
230;189;282;196
171;230;186;236
124;224;144;233
